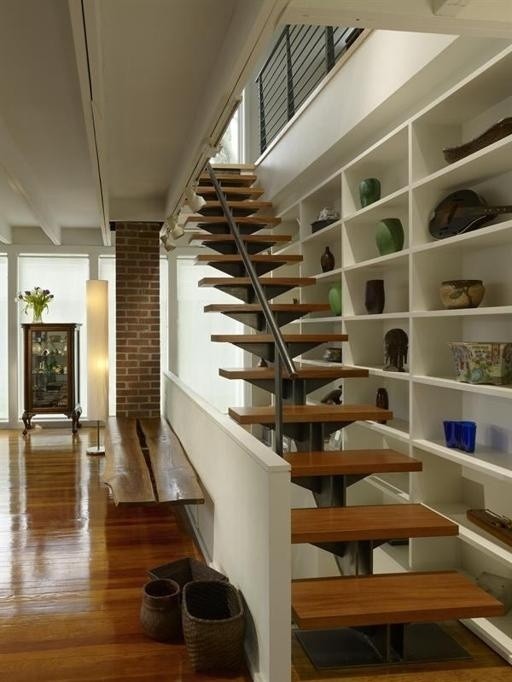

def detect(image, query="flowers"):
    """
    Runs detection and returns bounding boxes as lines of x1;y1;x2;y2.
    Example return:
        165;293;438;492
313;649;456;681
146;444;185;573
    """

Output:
14;286;54;319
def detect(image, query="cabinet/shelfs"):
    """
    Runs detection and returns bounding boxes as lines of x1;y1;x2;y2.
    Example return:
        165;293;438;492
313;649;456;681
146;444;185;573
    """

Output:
19;321;84;436
258;48;510;664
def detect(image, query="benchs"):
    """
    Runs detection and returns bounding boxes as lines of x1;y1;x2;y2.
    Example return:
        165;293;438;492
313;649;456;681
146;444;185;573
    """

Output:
102;413;206;547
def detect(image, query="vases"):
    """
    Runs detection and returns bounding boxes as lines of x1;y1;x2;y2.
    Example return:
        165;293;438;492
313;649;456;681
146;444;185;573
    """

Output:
359;178;381;208
321;244;335;272
374;385;390;426
329;288;344;317
365;279;384;314
374;216;405;256
31;304;45;325
436;279;486;308
138;576;182;644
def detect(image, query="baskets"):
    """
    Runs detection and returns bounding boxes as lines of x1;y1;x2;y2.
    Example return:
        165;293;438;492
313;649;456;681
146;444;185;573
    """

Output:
181;579;244;666
147;556;227;597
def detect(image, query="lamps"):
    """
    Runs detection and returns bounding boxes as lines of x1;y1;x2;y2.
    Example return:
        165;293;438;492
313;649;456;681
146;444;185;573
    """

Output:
84;279;111;457
159;180;207;252
